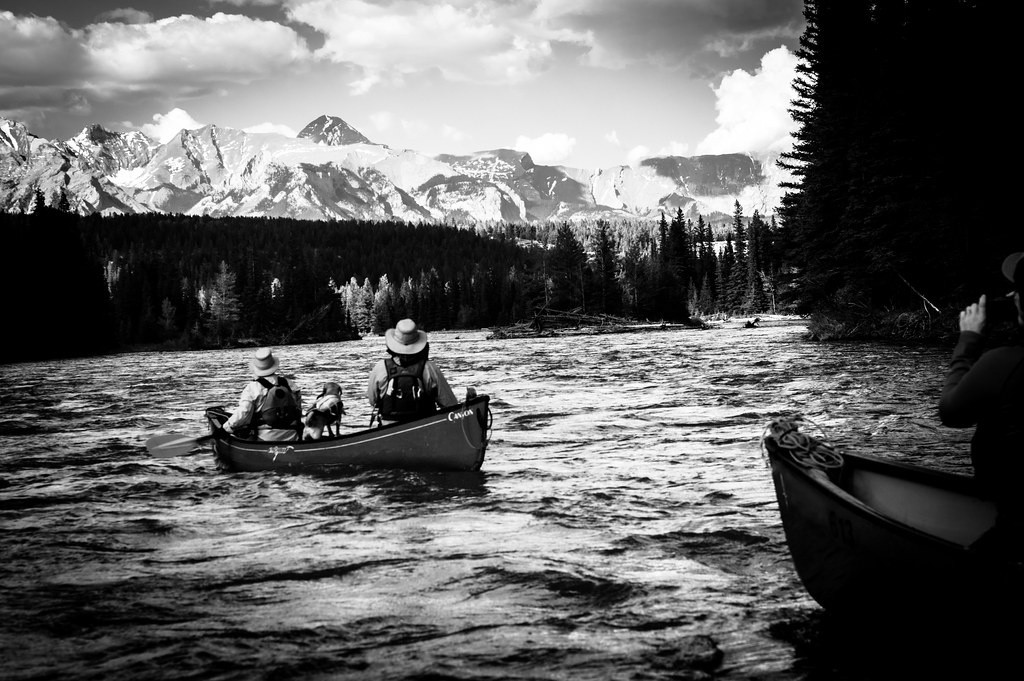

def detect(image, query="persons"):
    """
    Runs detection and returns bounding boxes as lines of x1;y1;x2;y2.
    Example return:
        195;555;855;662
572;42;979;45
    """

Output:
938;250;1024;561
211;347;302;442
367;318;458;427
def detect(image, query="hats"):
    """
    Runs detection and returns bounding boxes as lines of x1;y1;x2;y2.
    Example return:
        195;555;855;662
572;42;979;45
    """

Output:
384;318;427;354
248;348;279;376
